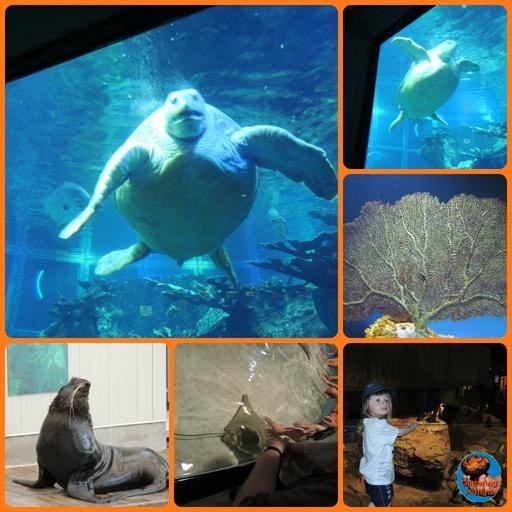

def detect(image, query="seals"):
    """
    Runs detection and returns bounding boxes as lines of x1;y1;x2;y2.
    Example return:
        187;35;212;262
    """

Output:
13;375;168;505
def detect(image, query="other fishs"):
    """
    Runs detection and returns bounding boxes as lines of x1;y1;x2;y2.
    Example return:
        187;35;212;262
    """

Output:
173;393;277;461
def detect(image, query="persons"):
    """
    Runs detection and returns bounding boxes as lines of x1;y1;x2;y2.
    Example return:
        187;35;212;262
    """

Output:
232;349;338;507
355;382;417;507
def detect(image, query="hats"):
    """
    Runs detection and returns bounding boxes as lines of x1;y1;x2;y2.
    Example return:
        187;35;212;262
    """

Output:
362;383;397;403
